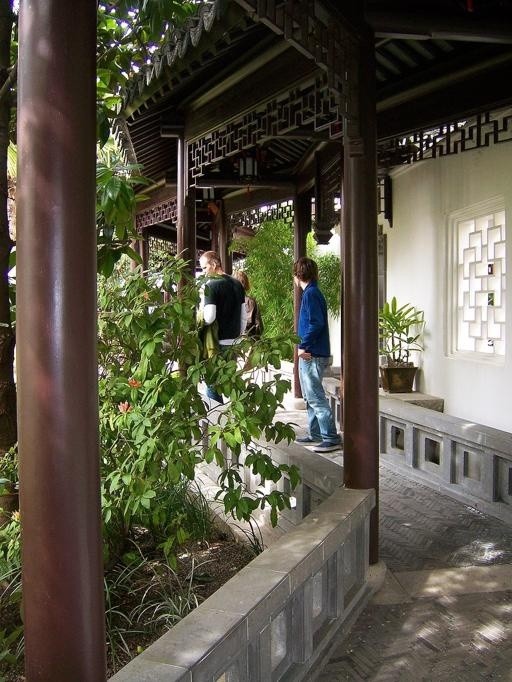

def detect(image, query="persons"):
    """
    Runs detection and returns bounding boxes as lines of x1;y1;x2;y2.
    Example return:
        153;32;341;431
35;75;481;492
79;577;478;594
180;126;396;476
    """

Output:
196;251;264;404
292;258;343;451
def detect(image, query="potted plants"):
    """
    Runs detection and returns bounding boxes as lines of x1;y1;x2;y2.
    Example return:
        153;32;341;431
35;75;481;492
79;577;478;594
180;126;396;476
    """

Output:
378;296;426;393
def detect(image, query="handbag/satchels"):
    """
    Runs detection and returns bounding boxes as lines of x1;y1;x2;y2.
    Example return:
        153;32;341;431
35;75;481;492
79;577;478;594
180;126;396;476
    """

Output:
244;301;264;342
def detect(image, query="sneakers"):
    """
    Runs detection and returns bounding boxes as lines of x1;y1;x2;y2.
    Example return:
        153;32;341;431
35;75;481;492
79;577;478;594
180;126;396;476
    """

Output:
313;442;342;453
294;437;319;445
